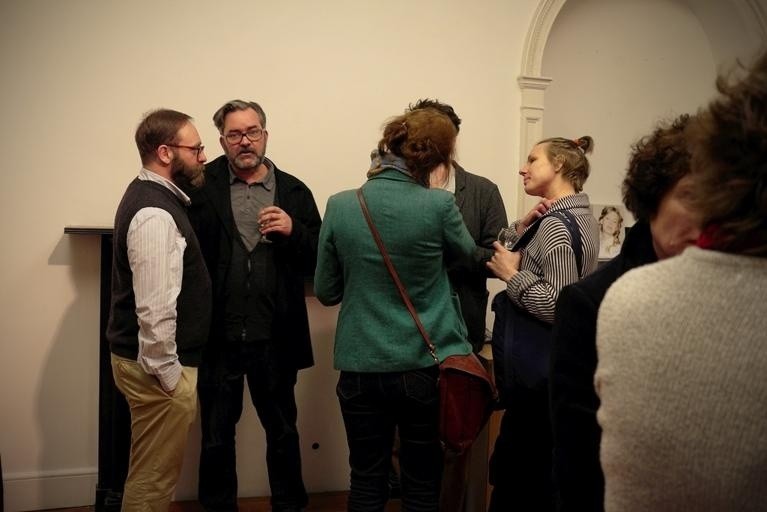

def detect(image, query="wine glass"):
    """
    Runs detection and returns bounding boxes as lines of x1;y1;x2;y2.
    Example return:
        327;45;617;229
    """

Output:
258;207;273;244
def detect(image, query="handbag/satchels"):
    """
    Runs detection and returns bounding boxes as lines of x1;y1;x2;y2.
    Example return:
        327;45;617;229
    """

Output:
491;290;573;410
439;352;500;459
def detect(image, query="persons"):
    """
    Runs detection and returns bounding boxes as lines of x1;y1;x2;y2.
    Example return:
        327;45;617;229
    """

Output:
312;106;478;510
550;111;703;512
485;134;602;512
186;100;322;512
406;98;509;360
598;206;623;258
103;107;215;511
595;44;765;512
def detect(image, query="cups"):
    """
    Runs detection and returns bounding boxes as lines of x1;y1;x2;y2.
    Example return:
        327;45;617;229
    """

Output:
498;228;521;251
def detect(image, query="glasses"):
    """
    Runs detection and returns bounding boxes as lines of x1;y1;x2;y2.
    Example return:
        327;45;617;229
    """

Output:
223;130;263;145
165;143;204;154
